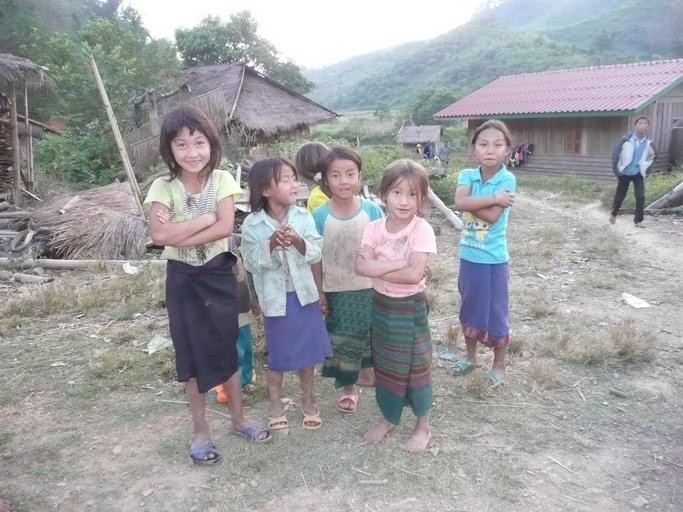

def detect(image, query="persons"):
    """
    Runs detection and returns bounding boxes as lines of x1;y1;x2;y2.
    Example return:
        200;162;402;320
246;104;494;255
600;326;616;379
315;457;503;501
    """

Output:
354;159;437;453
312;146;385;413
295;142;330;211
241;156;324;431
509;144;535;168
608;116;656;228
143;105;274;466
217;233;261;404
449;119;517;386
416;140;449;164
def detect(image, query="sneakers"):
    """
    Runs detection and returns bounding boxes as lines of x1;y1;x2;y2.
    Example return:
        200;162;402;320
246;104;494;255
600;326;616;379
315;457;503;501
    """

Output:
608;217;646;228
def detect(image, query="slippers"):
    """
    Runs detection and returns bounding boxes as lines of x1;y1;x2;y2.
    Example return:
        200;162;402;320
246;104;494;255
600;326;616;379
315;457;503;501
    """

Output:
303;406;322;429
233;426;272;442
189;443;222;464
336;395;359;413
450;360;473;374
482;372;503;388
267;415;288;430
